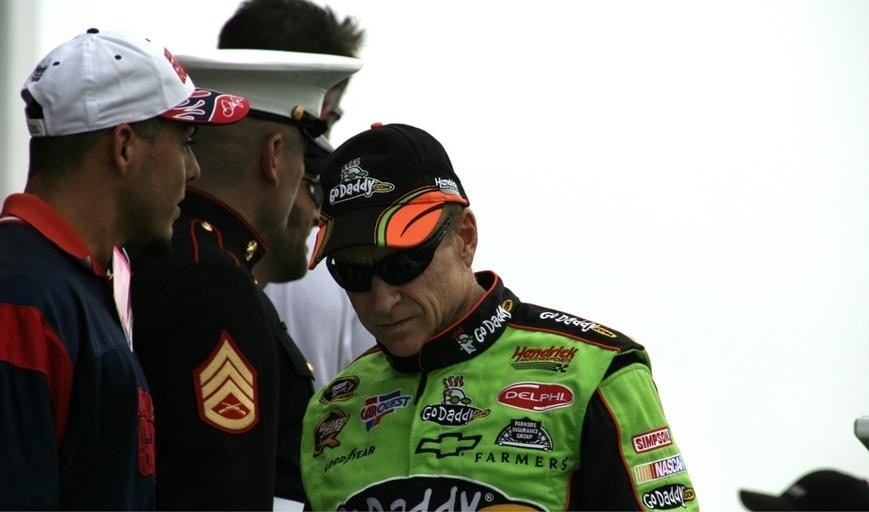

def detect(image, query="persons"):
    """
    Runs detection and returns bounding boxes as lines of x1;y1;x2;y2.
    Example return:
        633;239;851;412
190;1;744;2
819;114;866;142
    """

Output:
216;3;380;512
298;116;703;511
0;23;255;512
236;140;329;293
117;46;364;512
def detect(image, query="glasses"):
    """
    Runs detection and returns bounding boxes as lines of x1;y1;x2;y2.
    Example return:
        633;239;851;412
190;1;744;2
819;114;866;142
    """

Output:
326;207;457;294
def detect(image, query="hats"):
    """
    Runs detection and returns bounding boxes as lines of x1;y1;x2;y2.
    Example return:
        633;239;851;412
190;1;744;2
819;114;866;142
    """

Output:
308;124;470;272
303;133;334;180
20;28;253;138
174;48;362;131
737;467;869;511
